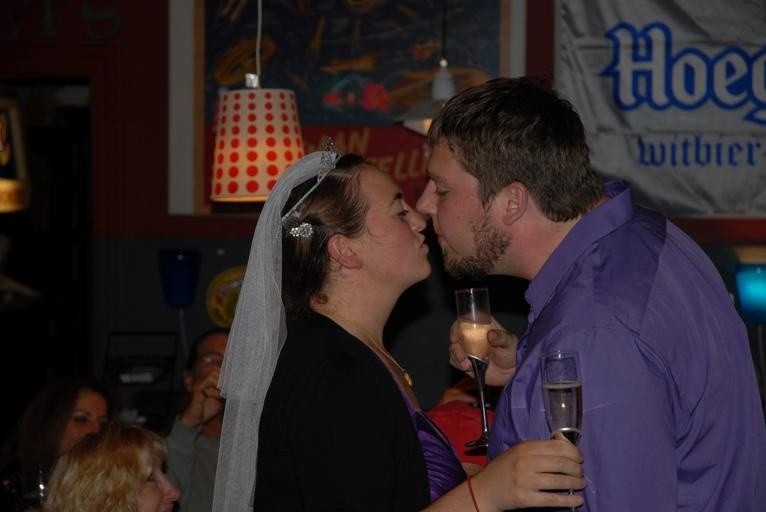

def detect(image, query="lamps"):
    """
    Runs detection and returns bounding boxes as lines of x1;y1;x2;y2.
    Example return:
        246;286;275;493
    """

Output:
210;1;308;202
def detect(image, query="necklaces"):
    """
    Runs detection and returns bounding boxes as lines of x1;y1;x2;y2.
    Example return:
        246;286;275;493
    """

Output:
355;326;414;386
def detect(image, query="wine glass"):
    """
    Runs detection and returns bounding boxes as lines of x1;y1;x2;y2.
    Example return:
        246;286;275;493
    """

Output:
450;286;499;450
539;347;585;512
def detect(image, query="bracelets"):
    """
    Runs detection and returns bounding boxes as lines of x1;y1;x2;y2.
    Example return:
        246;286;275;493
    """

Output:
467;475;481;512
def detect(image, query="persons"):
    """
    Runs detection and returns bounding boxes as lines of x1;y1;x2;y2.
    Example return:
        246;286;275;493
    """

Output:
414;71;766;511
212;133;589;511
2;373;122;512
417;371;501;466
41;416;182;512
152;328;232;511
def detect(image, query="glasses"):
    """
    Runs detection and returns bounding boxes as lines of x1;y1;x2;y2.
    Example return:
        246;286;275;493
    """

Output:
187;351;224;370
